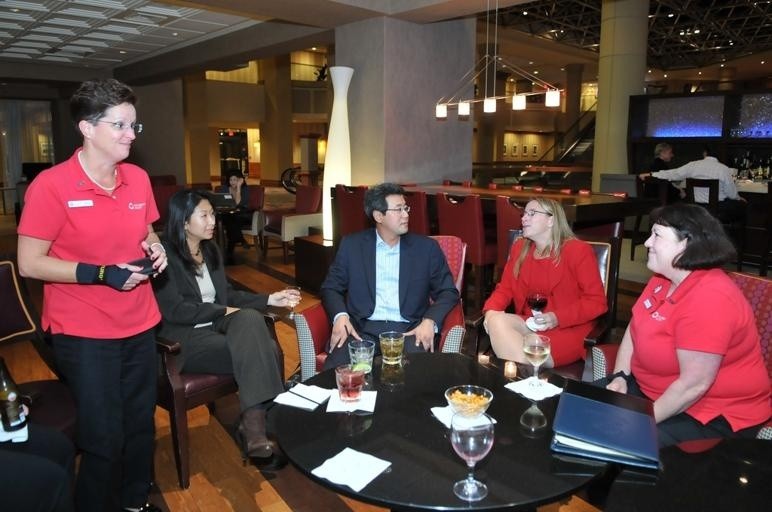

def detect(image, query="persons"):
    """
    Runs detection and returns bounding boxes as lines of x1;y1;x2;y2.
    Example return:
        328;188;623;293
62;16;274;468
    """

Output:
218;170;251;266
17;77;171;511
644;142;672;173
482;196;609;369
584;205;772;447
319;180;460;374
639;143;751;250
144;187;303;471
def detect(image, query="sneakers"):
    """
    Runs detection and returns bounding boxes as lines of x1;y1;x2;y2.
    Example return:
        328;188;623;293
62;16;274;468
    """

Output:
125;503;161;512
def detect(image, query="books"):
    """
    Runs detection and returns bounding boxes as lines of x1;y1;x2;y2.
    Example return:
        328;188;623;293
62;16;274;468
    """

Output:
549;393;660;471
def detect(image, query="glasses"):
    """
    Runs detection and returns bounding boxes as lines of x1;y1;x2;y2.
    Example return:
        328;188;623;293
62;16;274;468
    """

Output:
86;118;144;134
523;210;552;217
386;206;411;213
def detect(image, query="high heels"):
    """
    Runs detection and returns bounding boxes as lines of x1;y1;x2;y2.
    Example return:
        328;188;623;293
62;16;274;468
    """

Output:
237;408;272;462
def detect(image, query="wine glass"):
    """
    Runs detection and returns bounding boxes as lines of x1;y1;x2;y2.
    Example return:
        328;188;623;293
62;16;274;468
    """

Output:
448;412;496;502
285;283;301;318
519;398;550;442
525;334;551;390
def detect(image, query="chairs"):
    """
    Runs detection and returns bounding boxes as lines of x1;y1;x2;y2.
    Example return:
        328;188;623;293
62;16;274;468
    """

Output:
291;233;469;383
215;185;265;255
263;185;323;265
601;174;657;260
684;175;739;267
0;254;86;458
146;174;213;226
505;237;613;381
129;282;281;489
656;271;772;507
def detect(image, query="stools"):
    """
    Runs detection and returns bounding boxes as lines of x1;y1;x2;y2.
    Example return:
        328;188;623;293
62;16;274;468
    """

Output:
327;179;628;316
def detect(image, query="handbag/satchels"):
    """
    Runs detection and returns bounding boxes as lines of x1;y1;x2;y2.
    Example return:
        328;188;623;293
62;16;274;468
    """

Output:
707;438;772;495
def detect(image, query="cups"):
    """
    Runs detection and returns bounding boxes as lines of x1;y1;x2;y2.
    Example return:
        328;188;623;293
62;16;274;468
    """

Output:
379;330;404;365
527;290;549;314
347;338;374;375
334;361;365;402
377;363;408;392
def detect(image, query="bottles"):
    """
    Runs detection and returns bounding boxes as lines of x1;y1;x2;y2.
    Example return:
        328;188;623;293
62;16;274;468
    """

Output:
1;356;27;431
732;150;770;182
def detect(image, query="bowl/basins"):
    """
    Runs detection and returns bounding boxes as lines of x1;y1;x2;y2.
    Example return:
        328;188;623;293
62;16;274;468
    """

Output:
444;384;494;418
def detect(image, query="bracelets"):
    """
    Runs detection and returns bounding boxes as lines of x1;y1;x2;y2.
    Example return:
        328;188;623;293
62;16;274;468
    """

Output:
151;243;167;253
607;370;631;387
649;170;652;177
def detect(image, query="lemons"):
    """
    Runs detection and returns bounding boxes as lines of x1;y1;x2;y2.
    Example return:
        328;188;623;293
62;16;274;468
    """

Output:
350;362;372;374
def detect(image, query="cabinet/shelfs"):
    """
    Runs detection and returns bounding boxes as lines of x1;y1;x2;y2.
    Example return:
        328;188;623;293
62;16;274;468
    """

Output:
291;231;338;296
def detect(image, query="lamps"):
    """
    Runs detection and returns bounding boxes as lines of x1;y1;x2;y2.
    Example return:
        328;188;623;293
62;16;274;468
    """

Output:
435;1;561;119
322;65;355;240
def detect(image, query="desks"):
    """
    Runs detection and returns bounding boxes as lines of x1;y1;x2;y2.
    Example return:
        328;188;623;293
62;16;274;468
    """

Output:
274;351;627;510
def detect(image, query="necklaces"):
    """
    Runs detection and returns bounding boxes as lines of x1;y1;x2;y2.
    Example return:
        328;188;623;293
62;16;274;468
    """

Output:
188;247;205;265
77;150;118;191
